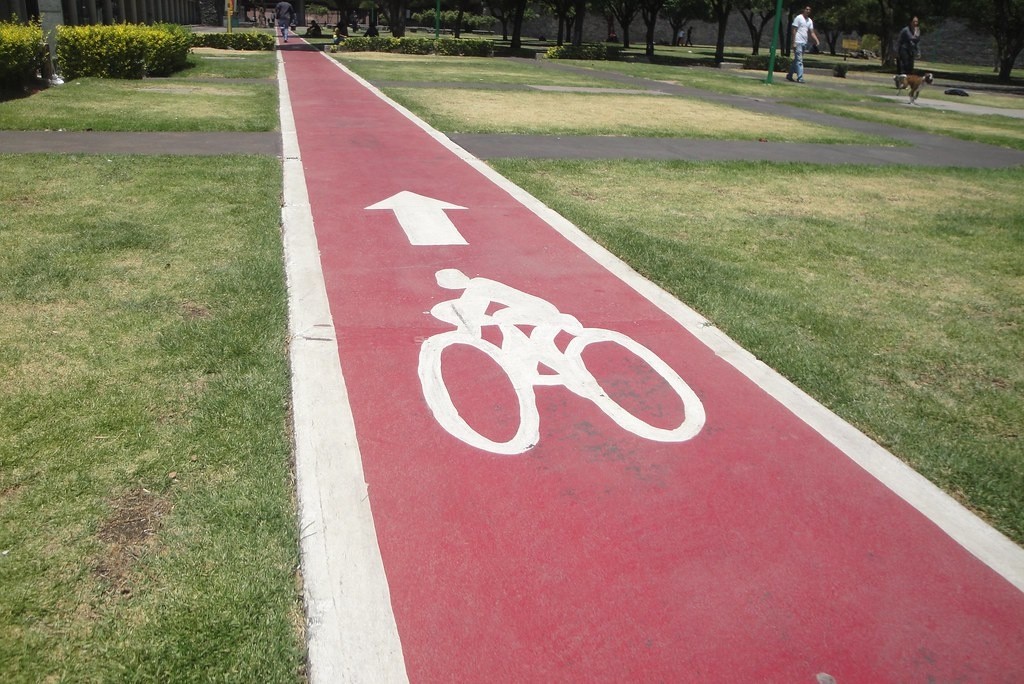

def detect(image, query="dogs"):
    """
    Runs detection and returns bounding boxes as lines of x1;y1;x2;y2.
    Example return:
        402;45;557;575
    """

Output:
893;71;933;105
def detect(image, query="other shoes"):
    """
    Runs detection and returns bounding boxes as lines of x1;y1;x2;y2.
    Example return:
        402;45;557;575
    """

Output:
786;74;795;81
796;77;805;83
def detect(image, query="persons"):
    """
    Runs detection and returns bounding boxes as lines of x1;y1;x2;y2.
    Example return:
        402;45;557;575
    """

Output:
894;14;921;75
253;0;378;46
786;4;820;83
808;43;819;54
609;26;694;47
